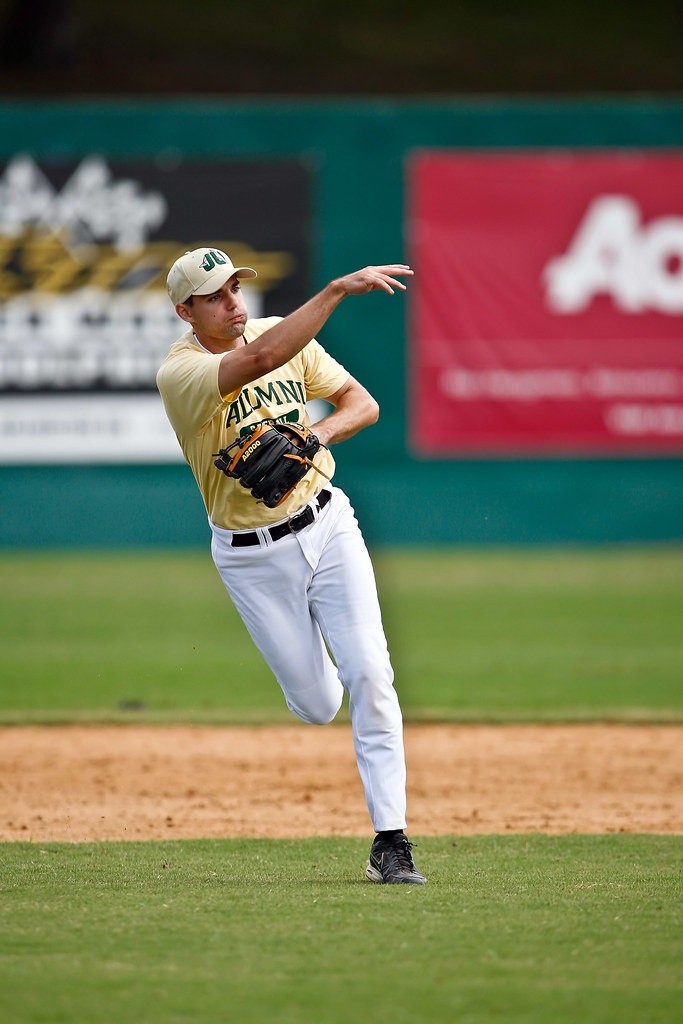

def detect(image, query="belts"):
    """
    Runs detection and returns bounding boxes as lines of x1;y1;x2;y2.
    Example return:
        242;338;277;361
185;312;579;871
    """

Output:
231;490;331;547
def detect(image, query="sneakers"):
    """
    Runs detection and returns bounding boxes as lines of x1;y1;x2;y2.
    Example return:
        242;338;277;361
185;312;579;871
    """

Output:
364;831;428;885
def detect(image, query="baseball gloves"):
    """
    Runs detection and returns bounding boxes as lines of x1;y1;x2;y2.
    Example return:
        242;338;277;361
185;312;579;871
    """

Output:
214;422;319;509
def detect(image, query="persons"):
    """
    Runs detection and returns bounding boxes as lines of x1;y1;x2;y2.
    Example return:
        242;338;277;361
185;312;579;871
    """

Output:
156;248;428;885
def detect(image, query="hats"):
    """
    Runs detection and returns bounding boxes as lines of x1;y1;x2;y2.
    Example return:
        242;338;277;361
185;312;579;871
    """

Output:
166;246;257;309
